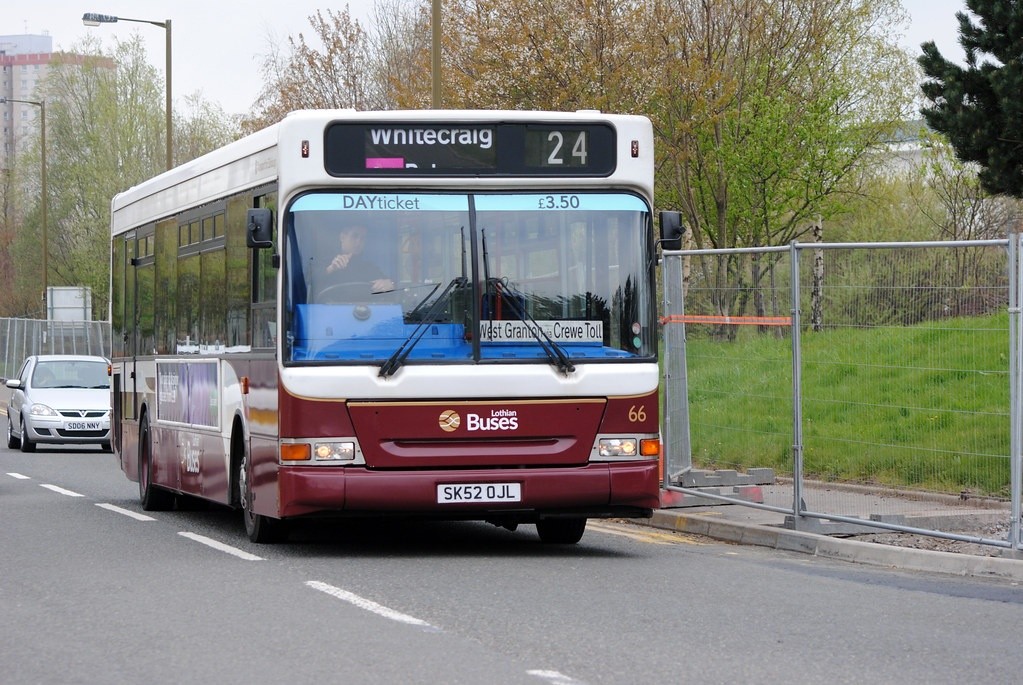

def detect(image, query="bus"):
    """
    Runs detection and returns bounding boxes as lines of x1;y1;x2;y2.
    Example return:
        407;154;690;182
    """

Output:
111;108;682;544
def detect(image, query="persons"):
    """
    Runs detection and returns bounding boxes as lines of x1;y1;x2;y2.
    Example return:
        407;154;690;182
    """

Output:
308;223;394;304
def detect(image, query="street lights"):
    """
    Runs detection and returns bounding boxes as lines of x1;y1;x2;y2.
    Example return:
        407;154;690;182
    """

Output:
0;97;47;332
82;14;170;175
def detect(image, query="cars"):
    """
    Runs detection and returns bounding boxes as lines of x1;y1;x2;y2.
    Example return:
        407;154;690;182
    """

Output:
6;355;113;453
